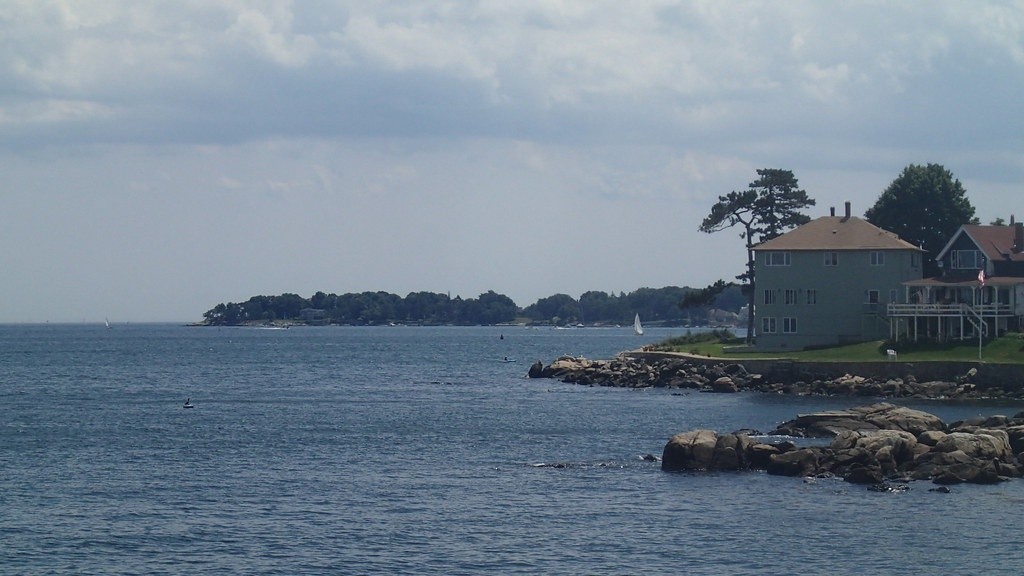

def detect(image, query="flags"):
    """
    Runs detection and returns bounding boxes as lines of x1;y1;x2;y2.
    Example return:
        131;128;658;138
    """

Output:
978;271;983;282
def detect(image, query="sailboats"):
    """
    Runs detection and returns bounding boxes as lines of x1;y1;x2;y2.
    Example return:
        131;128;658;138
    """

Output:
632;312;644;336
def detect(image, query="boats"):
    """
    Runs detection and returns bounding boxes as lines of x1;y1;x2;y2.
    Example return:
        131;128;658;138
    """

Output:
564;324;571;328
183;404;194;409
576;324;585;328
683;324;690;328
